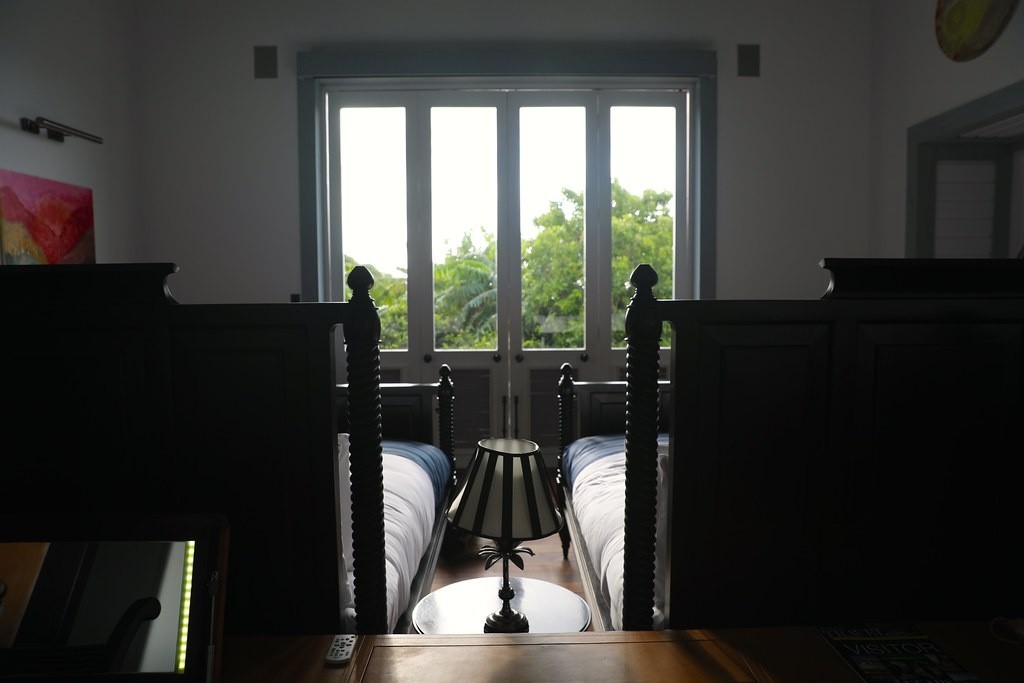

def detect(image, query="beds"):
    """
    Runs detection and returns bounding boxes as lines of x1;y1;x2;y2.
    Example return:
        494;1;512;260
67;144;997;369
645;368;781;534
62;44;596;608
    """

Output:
0;261;461;634
560;256;1024;633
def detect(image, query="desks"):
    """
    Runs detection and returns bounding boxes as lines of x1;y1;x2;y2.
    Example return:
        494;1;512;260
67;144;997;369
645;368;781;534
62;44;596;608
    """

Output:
218;612;1024;683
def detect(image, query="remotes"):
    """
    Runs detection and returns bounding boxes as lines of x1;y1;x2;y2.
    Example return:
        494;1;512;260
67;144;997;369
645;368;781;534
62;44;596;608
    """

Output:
326;634;358;666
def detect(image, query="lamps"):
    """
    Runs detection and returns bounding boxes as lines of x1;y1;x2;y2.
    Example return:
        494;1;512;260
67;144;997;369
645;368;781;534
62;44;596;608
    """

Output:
444;435;566;634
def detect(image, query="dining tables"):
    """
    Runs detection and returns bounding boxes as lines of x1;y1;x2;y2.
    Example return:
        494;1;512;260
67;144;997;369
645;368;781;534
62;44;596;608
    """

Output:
411;575;594;632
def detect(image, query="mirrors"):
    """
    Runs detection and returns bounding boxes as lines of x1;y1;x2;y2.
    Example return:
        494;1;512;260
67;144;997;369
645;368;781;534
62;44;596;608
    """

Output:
0;508;233;682
